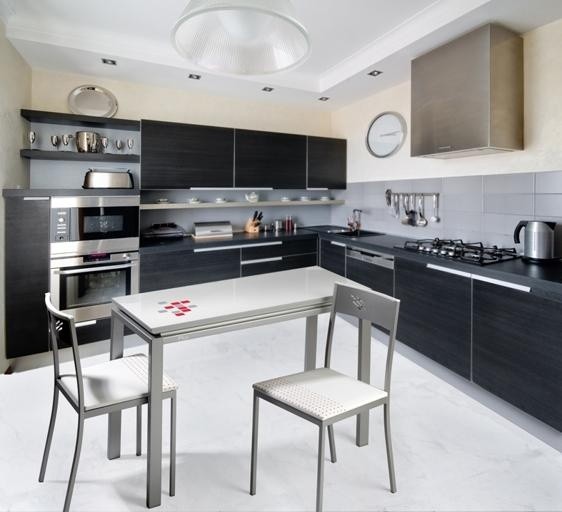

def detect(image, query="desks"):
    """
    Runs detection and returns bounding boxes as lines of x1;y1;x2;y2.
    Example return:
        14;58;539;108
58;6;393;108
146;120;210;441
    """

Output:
107;265;373;509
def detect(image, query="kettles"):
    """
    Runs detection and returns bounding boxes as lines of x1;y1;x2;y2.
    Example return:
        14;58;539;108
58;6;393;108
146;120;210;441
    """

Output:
514;219;557;265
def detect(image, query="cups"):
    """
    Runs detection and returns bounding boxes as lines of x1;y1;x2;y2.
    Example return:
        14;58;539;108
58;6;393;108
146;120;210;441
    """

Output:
155;192;331;204
264;214;294;230
76;132;102;153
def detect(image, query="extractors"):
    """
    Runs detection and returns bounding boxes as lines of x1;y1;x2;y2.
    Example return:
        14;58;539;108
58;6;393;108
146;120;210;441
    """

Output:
410;25;524;160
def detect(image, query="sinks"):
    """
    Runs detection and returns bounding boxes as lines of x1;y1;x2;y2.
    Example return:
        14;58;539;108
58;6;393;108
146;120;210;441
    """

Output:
336;232;384;238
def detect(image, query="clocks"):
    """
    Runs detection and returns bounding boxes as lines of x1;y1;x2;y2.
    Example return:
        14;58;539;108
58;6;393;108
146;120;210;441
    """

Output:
365;111;407;159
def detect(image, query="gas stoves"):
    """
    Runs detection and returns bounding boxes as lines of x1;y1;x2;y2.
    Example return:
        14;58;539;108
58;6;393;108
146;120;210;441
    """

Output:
392;237;515;265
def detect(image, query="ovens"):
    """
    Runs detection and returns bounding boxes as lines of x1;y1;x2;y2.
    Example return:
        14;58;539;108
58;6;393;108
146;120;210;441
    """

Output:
49;262;140;367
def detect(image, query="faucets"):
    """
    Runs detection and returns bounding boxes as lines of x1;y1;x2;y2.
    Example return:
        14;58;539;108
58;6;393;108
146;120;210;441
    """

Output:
348;210;361;231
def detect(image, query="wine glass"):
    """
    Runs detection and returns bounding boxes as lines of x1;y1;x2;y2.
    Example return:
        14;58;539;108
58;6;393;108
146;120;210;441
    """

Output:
127;139;134;156
27;131;35;150
51;136;59;151
101;137;109;154
62;135;69;151
116;140;123;155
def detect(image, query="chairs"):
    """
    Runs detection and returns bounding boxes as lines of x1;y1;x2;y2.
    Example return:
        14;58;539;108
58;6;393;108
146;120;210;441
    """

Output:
248;282;403;512
38;291;178;512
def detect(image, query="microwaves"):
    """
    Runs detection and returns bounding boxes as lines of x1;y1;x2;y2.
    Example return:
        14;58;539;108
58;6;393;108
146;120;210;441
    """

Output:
51;196;141;258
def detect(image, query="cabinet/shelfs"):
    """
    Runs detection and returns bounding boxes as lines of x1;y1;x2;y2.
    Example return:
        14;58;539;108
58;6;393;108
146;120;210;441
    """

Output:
344;246;394;336
136;233;319;293
318;238;345;276
18;109;140;162
139;118;348;190
393;254;562;435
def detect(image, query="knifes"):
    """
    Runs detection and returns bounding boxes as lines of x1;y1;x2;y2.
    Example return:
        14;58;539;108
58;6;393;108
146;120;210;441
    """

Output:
253;211;263;227
